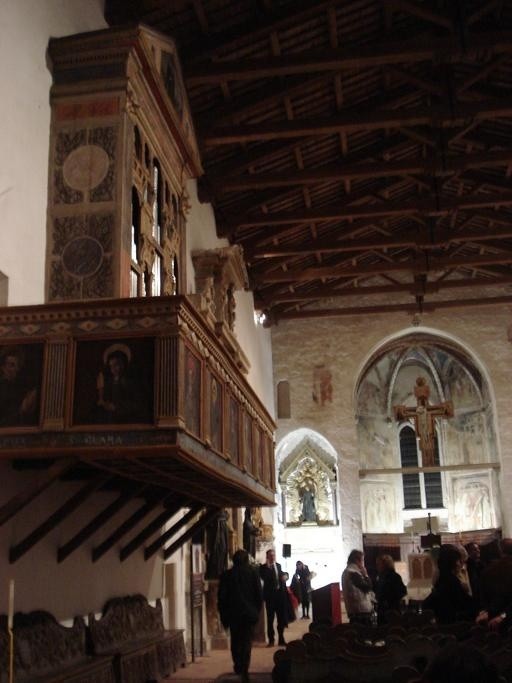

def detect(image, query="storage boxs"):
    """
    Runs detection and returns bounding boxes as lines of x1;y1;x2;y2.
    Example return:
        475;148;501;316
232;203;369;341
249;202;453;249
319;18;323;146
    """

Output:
274;599;511;683
0;610;115;682
89;591;186;683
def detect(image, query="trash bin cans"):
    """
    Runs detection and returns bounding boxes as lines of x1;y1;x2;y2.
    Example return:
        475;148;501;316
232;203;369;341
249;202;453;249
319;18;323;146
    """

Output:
500;613;506;619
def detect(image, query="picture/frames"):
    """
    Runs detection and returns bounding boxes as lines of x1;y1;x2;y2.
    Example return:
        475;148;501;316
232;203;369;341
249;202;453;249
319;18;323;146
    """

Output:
404;552;437;587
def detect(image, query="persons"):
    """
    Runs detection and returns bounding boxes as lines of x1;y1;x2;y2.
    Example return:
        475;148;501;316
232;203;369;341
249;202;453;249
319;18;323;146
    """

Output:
88;343;138;421
463;541;480;575
482;537;511;587
1;344;40;423
216;548;264;683
421;543;475;619
475;587;512;633
375;552;408;624
289;559;312;620
256;548;296;648
341;550;375;624
